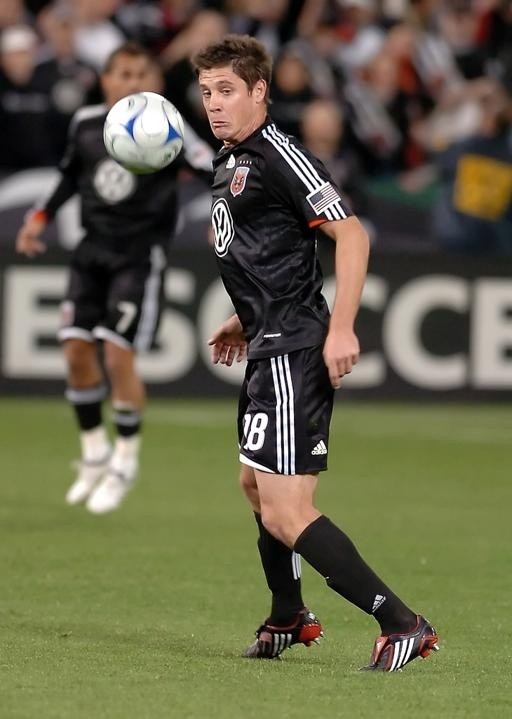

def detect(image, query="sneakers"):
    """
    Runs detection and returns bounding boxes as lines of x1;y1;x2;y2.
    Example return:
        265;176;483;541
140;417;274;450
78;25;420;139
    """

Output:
85;471;140;515
242;607;322;660
358;614;440;672
66;448;109;506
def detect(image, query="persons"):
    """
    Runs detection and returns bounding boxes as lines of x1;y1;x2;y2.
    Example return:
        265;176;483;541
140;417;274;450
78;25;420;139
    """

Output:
12;41;223;515
2;3;511;255
191;34;440;675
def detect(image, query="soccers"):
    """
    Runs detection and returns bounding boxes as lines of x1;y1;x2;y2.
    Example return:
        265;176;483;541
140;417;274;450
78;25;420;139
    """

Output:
103;91;183;174
